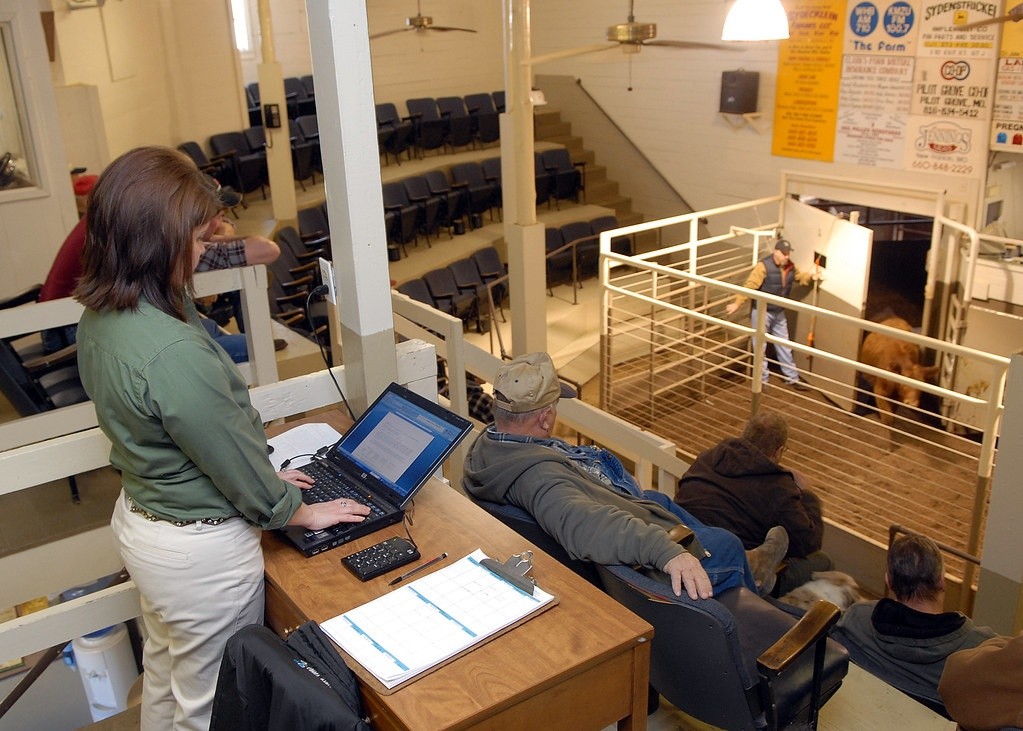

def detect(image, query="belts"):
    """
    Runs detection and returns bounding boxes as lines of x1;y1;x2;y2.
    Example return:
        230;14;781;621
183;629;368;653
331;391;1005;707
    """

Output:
123;490;260;528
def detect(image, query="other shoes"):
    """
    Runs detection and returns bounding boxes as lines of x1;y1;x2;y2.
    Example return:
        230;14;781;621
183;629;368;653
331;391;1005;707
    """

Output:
743;526;790;597
276;338;288;351
793;381;811;391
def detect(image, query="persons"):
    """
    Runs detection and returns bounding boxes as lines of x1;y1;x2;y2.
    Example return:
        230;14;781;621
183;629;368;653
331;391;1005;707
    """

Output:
726;239;823;391
42;174;289;383
840;533;1005;703
940;624;1023;731
464;351;789;601
74;144;372;731
674;413;834;601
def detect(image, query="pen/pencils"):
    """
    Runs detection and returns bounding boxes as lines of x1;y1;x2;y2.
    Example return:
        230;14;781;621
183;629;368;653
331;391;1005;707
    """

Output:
387;552;448;586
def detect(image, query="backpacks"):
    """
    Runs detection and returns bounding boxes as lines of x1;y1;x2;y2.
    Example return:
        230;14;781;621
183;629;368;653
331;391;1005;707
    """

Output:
285;618;378;731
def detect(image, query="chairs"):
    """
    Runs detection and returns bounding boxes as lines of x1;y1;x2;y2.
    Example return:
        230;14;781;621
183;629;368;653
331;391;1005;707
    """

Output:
0;282;92;505
459;479;849;731
178;74;631;334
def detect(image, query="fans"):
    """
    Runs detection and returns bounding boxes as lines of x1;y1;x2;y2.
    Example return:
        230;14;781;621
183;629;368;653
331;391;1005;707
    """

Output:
367;0;477;40
517;0;746;69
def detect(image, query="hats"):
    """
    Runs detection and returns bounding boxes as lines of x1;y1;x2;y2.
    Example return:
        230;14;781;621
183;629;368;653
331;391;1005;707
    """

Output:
494;352;577;413
776;240;795;252
202;171;243;208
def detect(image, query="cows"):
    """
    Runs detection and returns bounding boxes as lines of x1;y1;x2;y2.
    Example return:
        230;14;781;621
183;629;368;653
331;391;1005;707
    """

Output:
859;304;941;454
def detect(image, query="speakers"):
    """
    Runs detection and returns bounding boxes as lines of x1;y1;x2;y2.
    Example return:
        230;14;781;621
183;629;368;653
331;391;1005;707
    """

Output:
720;71;761;114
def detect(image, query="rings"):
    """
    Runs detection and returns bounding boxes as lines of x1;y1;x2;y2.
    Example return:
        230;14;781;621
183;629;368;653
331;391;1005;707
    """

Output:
342;502;347;506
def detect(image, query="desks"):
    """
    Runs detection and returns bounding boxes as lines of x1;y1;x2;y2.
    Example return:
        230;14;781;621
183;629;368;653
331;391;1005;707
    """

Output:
261;409;654;731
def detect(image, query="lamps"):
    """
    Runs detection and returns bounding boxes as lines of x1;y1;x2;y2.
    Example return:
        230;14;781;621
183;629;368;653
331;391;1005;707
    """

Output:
720;0;790;41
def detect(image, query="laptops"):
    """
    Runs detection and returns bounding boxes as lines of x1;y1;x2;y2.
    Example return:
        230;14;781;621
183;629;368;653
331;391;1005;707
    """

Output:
269;381;474;557
